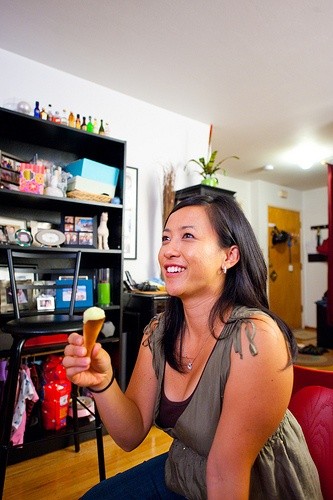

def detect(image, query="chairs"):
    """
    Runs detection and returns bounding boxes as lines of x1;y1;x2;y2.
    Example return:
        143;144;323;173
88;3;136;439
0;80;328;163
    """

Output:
289;384;333;500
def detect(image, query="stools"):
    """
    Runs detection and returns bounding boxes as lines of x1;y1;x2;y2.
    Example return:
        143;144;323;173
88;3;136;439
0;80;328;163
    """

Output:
0;244;110;499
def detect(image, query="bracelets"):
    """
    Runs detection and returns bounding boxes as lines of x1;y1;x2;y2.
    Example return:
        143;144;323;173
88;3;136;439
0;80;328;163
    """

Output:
85;372;114;393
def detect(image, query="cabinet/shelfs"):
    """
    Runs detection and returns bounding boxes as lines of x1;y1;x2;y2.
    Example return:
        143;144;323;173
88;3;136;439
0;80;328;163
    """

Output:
0;105;126;465
121;286;170;390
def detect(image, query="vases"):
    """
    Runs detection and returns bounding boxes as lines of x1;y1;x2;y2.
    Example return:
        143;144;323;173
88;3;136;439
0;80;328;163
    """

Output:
200;178;218;188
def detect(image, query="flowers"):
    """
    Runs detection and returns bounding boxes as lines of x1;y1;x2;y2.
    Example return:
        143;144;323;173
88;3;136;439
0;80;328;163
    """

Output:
184;122;240;181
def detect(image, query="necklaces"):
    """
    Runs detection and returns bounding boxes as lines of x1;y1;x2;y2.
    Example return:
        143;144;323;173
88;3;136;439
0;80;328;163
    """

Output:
182;320;220;370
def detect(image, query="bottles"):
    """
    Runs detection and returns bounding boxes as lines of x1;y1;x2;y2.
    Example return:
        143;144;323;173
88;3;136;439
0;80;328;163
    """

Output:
32;101;110;137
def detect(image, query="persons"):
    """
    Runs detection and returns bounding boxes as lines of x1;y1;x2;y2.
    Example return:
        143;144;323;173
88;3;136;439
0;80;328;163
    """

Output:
61;196;322;500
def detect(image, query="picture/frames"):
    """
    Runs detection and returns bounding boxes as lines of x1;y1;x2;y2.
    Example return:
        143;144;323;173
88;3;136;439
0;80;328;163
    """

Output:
123;165;137;260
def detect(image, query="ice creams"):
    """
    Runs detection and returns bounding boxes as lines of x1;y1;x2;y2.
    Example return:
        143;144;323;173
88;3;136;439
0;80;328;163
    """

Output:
81;306;105;364
